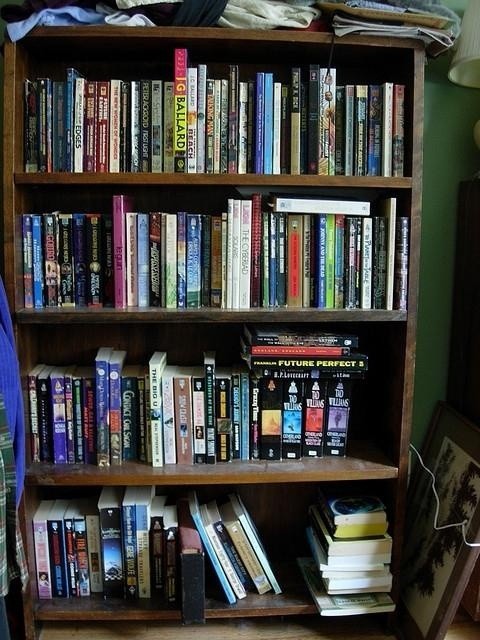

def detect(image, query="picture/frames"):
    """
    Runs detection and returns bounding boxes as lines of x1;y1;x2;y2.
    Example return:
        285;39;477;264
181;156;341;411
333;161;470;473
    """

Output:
395;400;480;640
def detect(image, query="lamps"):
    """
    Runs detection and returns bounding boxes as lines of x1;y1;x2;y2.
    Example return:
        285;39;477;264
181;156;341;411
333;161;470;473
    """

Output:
447;1;480;180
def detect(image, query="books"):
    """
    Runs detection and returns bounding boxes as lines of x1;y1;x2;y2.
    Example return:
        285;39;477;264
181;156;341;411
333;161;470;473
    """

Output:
23;194;409;312
27;329;370;467
296;491;398;618
31;484;282;626
20;46;407;179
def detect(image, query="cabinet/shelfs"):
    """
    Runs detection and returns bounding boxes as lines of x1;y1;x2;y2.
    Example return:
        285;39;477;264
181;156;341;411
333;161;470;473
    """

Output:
0;25;425;640
